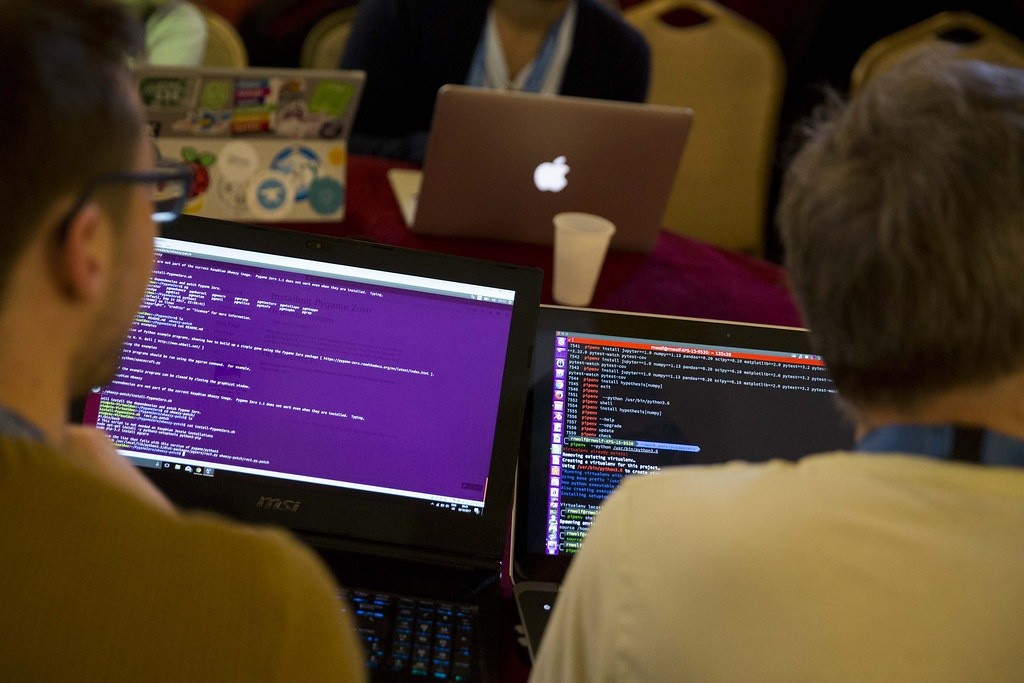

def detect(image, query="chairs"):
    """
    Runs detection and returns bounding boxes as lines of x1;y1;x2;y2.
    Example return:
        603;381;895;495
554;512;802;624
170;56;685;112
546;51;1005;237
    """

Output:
196;0;1023;262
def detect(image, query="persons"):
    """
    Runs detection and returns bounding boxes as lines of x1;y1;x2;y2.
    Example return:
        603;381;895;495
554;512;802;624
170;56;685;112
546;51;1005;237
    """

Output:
0;0;367;683
527;41;1024;683
347;0;652;167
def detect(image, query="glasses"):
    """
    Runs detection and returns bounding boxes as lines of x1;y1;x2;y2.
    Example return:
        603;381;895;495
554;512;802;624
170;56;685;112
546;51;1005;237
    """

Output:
57;161;192;245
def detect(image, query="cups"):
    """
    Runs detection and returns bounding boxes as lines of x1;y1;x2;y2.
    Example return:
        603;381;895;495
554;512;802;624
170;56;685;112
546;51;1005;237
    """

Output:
552;212;616;308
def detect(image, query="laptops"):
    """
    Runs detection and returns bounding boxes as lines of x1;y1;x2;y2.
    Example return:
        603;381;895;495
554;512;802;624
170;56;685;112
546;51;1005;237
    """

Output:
68;213;542;683
387;84;694;253
509;304;856;666
113;65;366;222
